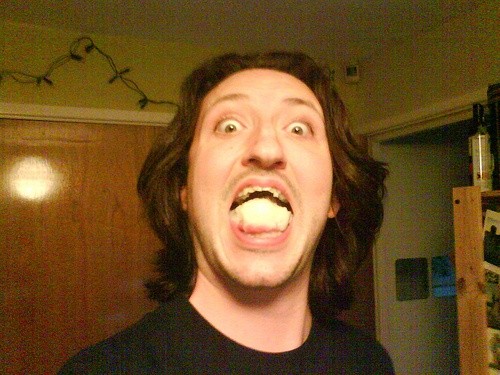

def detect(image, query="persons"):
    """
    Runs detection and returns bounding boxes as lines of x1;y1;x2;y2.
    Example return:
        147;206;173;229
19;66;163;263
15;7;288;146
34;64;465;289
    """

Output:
57;53;394;375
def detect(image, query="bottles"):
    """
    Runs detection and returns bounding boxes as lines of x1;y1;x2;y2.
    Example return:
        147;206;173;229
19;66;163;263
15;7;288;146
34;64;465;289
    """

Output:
468;104;493;191
